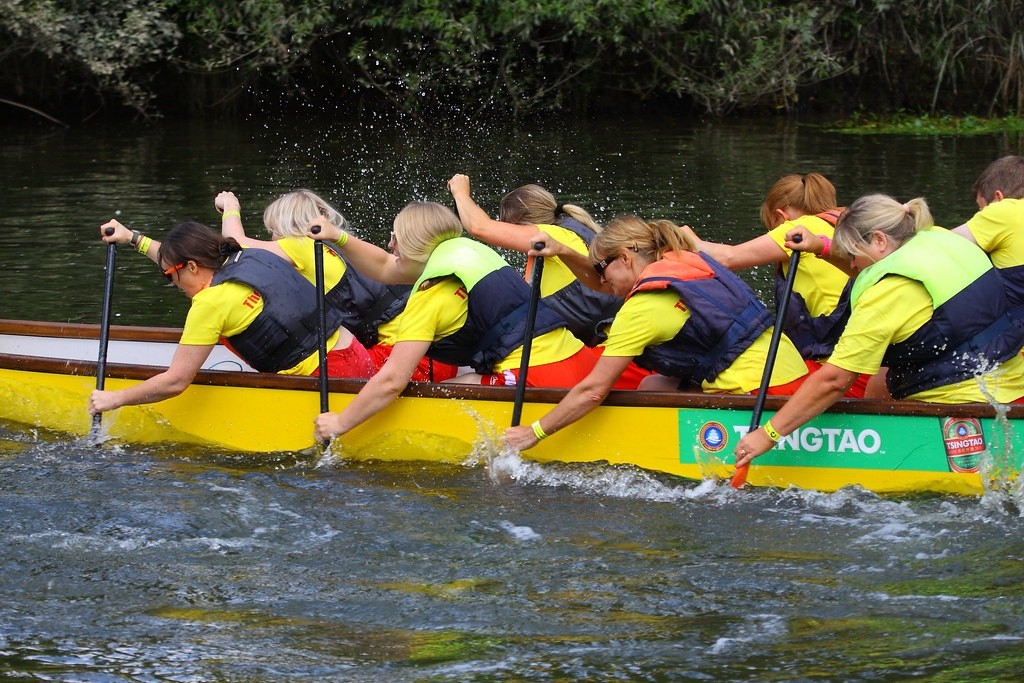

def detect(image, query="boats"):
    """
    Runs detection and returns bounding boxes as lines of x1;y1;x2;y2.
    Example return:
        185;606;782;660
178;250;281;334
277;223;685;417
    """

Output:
0;321;1024;502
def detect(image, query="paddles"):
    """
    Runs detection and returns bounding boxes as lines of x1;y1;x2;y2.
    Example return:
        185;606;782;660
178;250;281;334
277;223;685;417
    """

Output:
732;232;803;489
90;227;119;436
508;239;546;426
311;222;333;452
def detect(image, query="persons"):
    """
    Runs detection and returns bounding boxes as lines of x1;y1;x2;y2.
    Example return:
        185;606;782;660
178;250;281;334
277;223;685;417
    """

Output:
678;172;872;399
503;214;811;455
214;191;459;383
947;154;1024;314
88;219;372;415
734;192;1024;467
306;201;599;441
446;174;626;348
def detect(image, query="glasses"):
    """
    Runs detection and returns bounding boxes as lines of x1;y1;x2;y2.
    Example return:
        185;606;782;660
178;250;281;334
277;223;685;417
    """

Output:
848;251;856;260
390;232;399;244
162;263;187;281
594;257;615;276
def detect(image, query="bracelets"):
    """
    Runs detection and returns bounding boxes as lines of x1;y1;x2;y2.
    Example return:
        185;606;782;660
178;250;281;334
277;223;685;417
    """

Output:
221;210;241;221
138;236;153;255
531;420;548;440
331;230;349;247
763;419;785;444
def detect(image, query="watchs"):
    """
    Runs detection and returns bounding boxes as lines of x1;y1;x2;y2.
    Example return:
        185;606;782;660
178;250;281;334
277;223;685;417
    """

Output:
128;229;140;249
